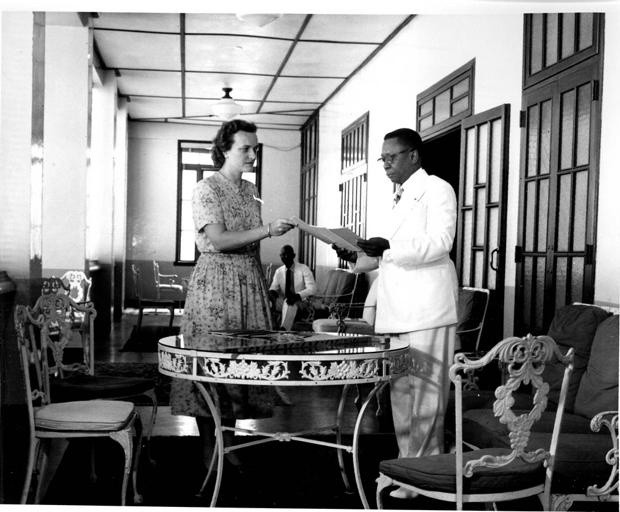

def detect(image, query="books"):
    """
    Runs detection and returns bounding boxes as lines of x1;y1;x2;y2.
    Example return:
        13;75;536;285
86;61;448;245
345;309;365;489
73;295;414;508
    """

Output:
289;214;365;254
207;326;280;338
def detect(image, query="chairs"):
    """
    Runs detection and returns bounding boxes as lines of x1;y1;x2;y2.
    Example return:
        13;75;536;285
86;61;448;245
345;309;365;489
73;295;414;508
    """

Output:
297;269;358;329
374;332;577;510
372;285;492;420
127;262;185;332
495;409;618;511
445;302;612;430
462;315;619;446
10;270;158;505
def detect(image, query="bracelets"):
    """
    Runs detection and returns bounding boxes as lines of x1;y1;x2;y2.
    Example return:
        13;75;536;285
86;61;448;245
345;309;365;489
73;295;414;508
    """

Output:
267;222;271;239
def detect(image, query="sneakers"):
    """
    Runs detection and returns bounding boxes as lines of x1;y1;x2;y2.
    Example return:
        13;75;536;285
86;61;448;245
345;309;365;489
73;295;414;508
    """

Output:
389;486;419;499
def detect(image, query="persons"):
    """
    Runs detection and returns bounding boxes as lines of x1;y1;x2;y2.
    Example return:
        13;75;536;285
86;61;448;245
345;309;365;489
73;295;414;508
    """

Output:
267;243;316;332
169;115;301;482
331;126;465;502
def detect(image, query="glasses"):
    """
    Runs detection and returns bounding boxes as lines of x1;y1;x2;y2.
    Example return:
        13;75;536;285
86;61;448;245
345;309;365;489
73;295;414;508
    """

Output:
377;148;410;166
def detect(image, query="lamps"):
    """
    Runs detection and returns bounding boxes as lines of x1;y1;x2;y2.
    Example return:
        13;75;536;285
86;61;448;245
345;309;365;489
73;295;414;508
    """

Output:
215;89;241;120
